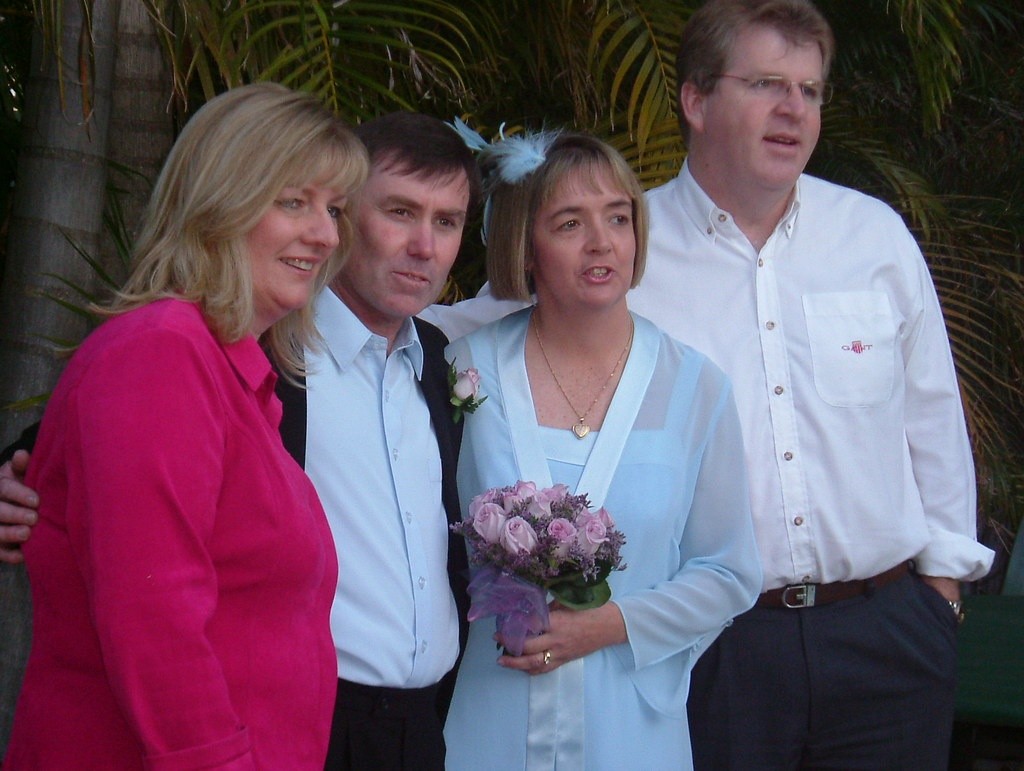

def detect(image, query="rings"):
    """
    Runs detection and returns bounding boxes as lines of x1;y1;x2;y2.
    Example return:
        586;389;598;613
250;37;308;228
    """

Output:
543;649;551;665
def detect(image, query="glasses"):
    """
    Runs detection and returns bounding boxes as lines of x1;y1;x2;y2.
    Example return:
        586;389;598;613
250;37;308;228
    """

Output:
710;73;834;105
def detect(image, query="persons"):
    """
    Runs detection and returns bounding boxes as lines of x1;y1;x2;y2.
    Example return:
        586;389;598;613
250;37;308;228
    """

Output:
415;0;995;771
443;116;762;771
0;81;370;771
0;111;488;771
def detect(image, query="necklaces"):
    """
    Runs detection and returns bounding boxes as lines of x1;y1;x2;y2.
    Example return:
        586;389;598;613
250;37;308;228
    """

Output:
533;309;632;440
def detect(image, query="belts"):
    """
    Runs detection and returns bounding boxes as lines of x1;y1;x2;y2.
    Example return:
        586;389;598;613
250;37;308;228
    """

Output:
751;562;908;613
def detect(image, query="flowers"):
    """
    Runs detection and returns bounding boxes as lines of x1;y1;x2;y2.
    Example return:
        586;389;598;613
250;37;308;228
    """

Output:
450;481;628;657
443;357;488;423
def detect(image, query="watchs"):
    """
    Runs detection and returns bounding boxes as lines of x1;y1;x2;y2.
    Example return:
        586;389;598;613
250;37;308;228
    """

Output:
948;600;966;625
